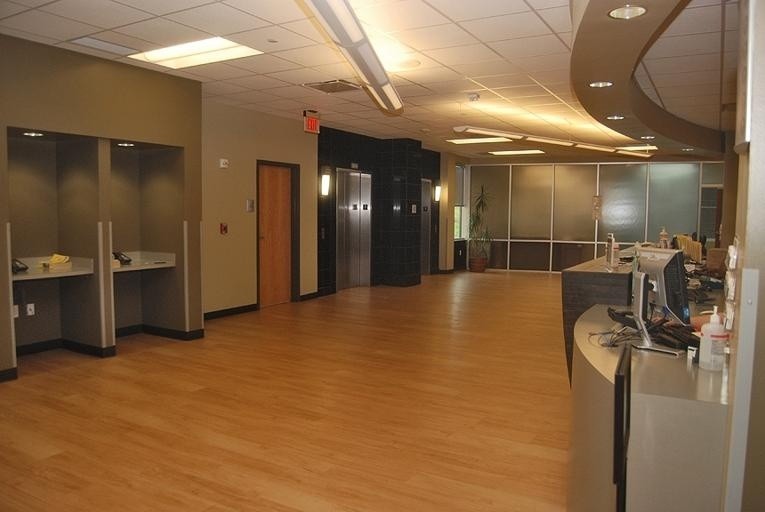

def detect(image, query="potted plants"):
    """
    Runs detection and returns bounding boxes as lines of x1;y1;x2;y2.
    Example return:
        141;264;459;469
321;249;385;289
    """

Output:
467;183;496;272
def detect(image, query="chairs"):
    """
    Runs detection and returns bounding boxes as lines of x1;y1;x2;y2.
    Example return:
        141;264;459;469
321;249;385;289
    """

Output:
689;247;727;306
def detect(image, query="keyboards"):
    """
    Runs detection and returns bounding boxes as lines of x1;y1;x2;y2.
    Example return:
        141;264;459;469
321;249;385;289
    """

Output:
658;326;700;347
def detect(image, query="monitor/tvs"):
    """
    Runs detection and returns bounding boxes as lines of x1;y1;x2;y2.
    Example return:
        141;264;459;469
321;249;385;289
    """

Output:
632;248;688;357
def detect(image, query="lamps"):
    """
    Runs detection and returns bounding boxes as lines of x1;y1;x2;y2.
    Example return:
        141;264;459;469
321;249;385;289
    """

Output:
298;1;404;118
451;102;656;158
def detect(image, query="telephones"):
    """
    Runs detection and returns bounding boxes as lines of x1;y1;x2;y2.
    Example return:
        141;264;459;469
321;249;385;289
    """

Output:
113;252;131;264
12;258;29;273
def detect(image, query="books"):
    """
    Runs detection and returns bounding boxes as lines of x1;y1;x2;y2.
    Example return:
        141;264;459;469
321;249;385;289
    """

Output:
35;253;72;273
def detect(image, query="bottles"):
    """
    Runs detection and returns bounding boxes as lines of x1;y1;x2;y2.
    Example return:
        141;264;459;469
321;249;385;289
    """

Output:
606;233;620;268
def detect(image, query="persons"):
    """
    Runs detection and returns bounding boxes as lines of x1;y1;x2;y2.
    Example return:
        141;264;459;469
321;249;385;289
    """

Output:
651;311;726;333
